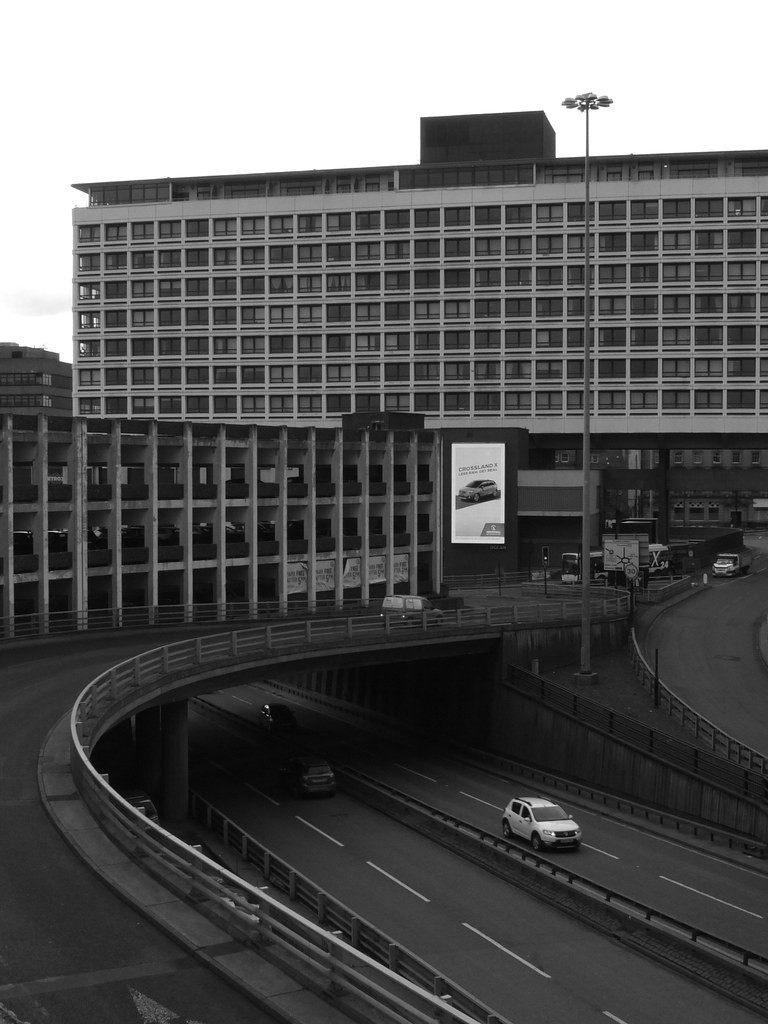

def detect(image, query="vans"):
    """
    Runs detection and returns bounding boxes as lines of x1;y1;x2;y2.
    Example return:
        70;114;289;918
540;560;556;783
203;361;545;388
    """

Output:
459;478;498;501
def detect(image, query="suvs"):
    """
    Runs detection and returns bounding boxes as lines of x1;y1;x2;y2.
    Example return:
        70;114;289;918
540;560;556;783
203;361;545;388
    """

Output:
502;796;584;853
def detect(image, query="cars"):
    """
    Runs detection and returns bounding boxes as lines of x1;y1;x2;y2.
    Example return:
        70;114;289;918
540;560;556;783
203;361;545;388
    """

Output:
382;596;444;628
292;756;335;800
258;703;298;732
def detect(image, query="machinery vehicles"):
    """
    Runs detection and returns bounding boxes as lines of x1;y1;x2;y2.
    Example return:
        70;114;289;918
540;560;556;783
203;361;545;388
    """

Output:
710;549;753;578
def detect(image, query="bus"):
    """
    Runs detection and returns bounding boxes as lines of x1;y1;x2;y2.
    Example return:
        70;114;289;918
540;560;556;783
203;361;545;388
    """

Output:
561;545;669;586
